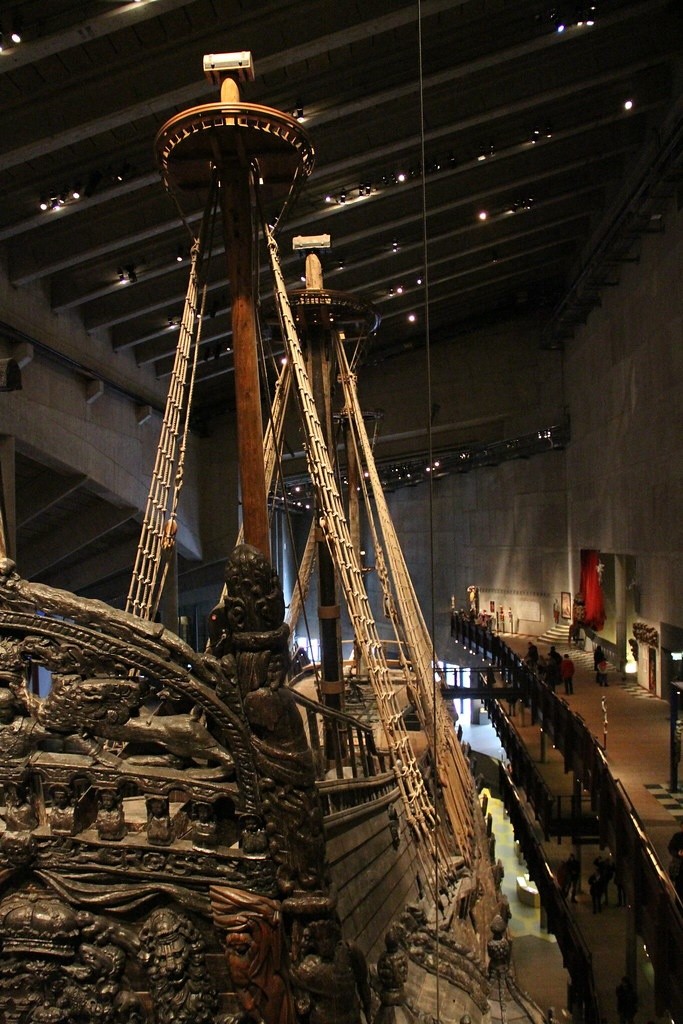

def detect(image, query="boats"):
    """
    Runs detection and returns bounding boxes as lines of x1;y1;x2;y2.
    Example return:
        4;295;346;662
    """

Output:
1;51;515;1024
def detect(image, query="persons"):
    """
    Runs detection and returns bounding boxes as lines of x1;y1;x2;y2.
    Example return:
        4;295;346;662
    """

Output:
293;904;490;1024
524;642;575;694
557;853;625;915
668;822;683;904
452;608;492;632
594;646;610;686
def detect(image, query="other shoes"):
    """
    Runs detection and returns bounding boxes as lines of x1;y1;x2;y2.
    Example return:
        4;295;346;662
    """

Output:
604;683;609;687
600;682;603;687
